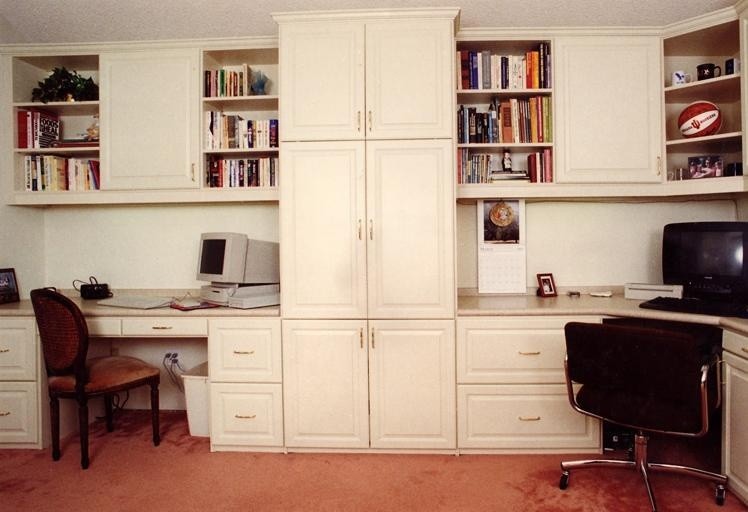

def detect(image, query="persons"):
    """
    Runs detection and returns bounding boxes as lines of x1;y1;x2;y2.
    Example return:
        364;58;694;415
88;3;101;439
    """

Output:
543;281;551;292
689;156;722;180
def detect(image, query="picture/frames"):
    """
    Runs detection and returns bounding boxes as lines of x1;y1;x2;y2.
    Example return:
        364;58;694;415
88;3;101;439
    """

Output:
535;272;559;298
0;267;20;305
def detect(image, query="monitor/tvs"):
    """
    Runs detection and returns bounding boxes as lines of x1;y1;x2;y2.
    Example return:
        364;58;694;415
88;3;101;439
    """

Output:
196;231;281;284
662;221;748;300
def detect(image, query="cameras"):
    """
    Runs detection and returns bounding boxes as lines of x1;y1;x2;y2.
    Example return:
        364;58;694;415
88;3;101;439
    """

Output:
81;283;109;300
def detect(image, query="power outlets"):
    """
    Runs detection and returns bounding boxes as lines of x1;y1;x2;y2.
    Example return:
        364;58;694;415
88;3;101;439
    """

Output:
166;349;181;365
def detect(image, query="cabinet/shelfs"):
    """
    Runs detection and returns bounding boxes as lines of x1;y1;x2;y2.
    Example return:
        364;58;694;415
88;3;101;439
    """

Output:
203;37;280;201
0;40;102;208
552;25;665;196
280;140;453;317
103;40;202;206
283;321;454;457
269;2;463;130
664;3;747;197
456;29;552;198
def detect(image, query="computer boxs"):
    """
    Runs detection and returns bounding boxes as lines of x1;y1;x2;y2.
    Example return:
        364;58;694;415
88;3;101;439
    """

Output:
199;284;281;309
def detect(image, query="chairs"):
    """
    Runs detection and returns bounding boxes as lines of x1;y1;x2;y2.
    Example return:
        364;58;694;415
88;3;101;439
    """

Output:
560;316;726;511
29;287;164;471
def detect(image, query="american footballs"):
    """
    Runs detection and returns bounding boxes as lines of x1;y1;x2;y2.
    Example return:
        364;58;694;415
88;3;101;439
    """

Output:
678;100;722;138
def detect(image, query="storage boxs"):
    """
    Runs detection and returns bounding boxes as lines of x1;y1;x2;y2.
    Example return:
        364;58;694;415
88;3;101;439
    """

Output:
180;359;209;439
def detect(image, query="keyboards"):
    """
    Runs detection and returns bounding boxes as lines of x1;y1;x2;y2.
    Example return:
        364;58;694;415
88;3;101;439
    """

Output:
639;297;745;317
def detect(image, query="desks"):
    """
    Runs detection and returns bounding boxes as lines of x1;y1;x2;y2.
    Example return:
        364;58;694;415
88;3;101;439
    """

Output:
454;289;748;506
1;289;283;455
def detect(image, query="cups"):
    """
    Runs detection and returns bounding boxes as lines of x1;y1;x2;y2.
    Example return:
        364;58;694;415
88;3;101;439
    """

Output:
670;70;693;86
696;62;722;81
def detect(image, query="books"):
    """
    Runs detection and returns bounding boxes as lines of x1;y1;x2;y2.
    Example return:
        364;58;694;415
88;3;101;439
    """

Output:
19;110;100;192
203;62;276;186
457;39;553;184
